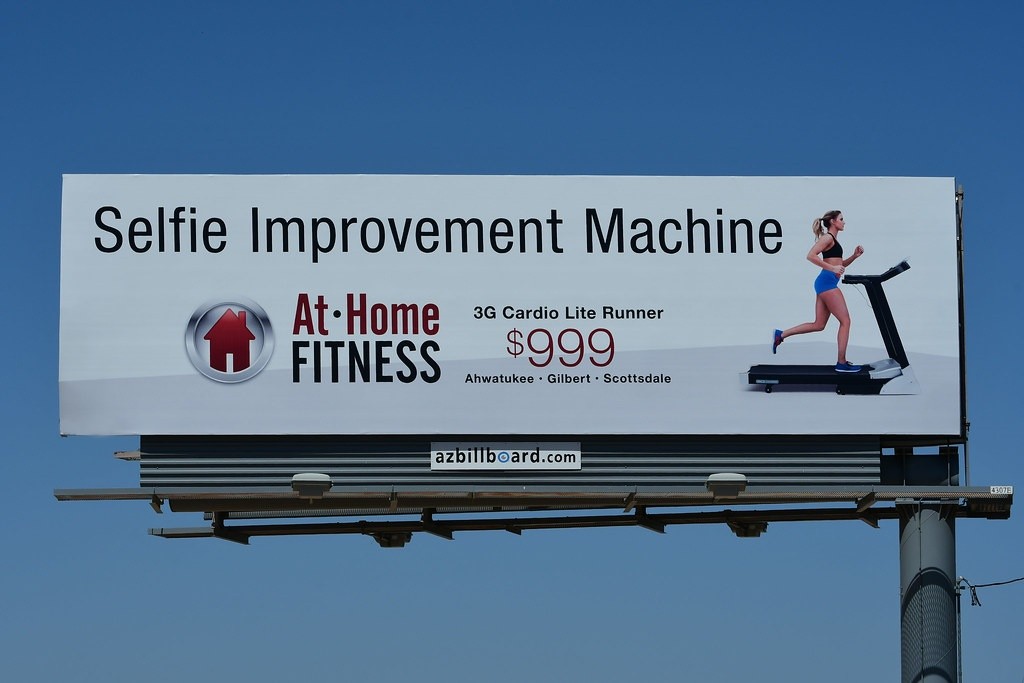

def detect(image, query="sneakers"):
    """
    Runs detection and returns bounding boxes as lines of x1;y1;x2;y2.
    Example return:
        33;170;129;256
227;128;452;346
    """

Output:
835;361;862;373
771;328;784;355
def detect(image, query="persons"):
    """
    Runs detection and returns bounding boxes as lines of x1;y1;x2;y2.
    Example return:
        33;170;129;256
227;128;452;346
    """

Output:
772;210;864;372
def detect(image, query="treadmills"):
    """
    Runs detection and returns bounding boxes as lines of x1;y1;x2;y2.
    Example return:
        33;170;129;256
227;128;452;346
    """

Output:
738;260;914;395
